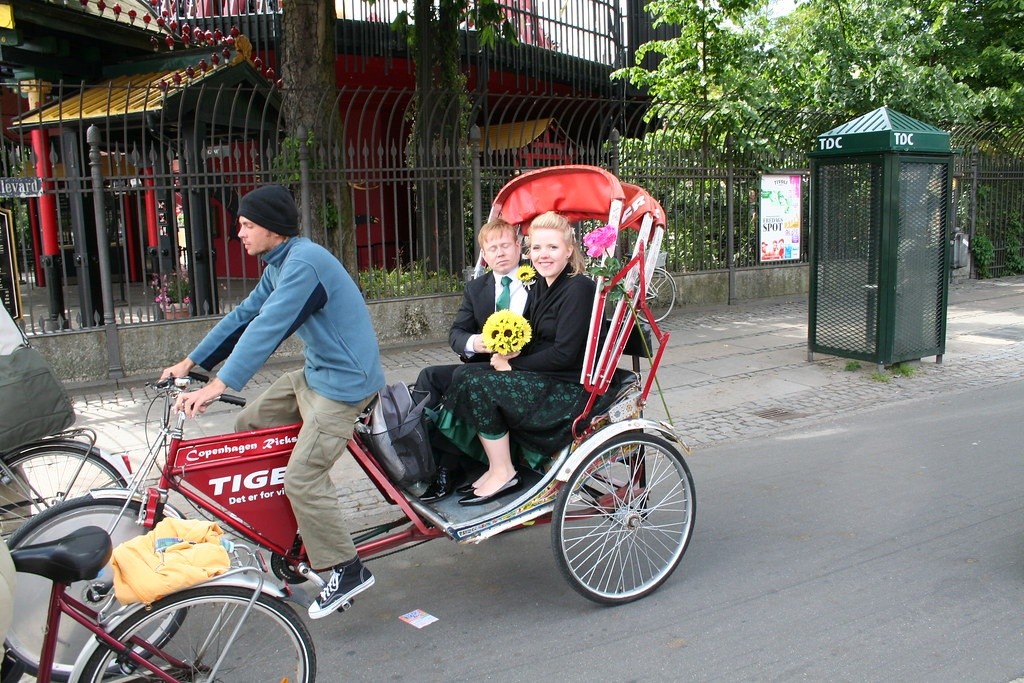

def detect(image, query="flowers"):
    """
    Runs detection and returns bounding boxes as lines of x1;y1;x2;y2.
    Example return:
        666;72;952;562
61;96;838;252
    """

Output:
516;264;536;290
482;309;532;355
584;225;625;301
152;270;195;304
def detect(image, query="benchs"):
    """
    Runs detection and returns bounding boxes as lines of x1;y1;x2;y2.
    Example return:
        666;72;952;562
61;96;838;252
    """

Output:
517;320;651;454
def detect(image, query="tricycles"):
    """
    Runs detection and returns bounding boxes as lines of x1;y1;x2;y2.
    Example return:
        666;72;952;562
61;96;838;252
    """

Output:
0;154;703;683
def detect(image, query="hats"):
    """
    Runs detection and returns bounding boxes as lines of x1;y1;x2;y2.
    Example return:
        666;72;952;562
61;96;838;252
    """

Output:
236;184;300;237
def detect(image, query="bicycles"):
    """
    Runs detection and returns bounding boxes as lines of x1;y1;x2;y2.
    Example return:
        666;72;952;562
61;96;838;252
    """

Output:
616;253;678;322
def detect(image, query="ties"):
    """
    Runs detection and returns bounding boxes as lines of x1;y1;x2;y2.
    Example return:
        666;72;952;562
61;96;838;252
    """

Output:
496;276;512;311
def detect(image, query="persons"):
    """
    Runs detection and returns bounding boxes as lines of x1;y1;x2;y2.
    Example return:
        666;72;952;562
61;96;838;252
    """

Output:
762;242;768;257
771;239;784;259
0;299;32;538
158;184;386;620
438;210;622;505
412;219;541;502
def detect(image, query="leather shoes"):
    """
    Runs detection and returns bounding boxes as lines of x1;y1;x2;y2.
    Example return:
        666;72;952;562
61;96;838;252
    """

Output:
456;465;519;495
458;471;522;505
420;465;459;504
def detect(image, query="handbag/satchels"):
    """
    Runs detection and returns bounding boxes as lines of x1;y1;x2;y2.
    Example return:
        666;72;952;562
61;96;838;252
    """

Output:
0;345;77;454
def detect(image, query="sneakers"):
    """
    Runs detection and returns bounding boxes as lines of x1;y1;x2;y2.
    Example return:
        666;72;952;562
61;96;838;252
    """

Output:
308;554;375;620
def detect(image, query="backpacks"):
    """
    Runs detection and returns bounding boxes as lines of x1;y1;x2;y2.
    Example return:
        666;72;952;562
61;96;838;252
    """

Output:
367;381;431;498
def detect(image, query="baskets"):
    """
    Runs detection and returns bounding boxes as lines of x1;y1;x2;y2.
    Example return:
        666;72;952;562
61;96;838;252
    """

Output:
645;249;668;268
358;402;438;486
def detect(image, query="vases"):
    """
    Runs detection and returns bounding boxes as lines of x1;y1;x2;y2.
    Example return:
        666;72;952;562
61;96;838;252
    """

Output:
160;302;189;319
